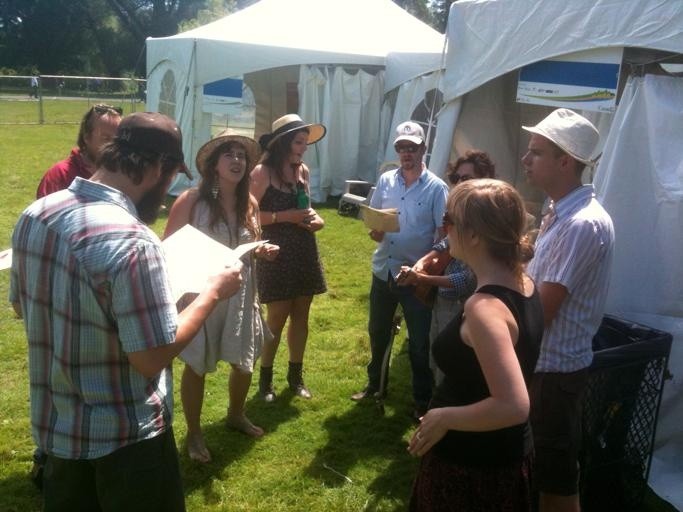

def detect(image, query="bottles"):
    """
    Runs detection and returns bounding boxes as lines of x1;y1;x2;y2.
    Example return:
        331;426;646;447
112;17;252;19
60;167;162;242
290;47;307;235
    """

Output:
392;121;426;153
521;105;604;168
112;110;194;183
256;112;326;151
195;126;264;177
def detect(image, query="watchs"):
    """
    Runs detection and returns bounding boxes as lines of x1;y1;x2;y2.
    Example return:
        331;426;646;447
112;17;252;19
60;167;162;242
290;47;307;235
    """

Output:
8;111;243;512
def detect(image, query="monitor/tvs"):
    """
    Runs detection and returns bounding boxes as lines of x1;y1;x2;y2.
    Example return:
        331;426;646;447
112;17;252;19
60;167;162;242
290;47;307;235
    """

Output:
162;223;271;317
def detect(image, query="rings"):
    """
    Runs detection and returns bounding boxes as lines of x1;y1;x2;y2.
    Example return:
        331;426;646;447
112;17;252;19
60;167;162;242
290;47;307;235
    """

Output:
416;432;421;440
304;217;308;223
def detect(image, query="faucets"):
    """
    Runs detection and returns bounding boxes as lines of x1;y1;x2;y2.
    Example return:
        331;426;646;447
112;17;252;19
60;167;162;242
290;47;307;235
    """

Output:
225;406;263;439
184;426;212;464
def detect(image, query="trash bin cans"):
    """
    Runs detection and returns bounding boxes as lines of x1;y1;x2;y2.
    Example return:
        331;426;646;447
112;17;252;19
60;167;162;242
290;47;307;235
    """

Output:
8;111;243;512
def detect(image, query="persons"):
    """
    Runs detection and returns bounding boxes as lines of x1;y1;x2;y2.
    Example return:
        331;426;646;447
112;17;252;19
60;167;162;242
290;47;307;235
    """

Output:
394;150;498;391
352;122;450;423
249;113;326;405
37;104;122;201
407;176;544;511
29;73;38;98
522;107;613;510
163;126;280;463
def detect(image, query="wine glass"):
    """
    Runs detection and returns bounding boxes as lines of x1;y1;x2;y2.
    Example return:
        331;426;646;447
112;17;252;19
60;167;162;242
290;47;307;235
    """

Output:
304;217;308;223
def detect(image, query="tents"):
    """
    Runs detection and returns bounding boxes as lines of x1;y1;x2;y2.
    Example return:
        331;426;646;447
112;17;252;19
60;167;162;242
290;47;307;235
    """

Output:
145;0;448;204
428;0;683;512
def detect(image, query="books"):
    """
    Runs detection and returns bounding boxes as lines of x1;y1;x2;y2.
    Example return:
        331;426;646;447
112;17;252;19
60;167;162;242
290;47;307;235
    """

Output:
162;223;271;317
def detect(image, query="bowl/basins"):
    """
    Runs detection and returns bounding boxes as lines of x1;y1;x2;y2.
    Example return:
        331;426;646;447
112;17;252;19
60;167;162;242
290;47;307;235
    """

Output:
272;211;277;225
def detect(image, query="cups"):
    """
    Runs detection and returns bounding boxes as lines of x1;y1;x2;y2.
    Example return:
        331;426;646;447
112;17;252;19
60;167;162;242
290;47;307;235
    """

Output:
447;170;472;183
85;104;123;125
394;144;421;153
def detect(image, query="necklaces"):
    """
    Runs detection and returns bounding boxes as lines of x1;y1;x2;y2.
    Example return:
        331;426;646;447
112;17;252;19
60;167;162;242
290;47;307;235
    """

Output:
272;211;277;225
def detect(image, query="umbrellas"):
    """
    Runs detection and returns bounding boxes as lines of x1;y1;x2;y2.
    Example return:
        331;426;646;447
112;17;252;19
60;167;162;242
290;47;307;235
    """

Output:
351;389;388;402
405;396;431;418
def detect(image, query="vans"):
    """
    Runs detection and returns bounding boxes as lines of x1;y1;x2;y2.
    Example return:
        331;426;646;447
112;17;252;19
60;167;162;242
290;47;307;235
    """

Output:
258;378;275;405
286;371;312;399
